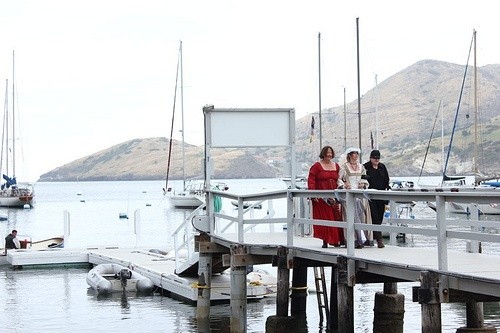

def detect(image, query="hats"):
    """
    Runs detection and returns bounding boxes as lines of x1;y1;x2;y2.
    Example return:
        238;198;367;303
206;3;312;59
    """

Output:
344;148;360;157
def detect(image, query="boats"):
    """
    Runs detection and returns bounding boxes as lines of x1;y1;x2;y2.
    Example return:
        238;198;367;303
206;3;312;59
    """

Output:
282;174;309;189
86;262;155;294
230;200;263;210
0;234;64;266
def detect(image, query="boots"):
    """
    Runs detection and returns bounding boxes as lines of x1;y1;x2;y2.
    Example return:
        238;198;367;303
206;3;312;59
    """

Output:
375;232;385;248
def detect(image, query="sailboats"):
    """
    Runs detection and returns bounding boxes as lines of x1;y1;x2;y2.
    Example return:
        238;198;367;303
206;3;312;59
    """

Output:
0;49;36;208
160;40;229;208
417;28;500;215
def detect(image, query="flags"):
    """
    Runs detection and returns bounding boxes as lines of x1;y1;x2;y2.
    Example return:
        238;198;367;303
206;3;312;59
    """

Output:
309;116;315;144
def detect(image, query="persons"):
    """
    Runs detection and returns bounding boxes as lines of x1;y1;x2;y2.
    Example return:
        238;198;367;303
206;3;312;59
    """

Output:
361;150;391;248
337;148;375;249
307;146;345;248
5;230;20;249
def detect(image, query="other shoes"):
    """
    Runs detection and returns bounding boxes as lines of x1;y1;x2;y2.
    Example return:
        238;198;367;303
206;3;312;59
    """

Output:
322;240;327;248
